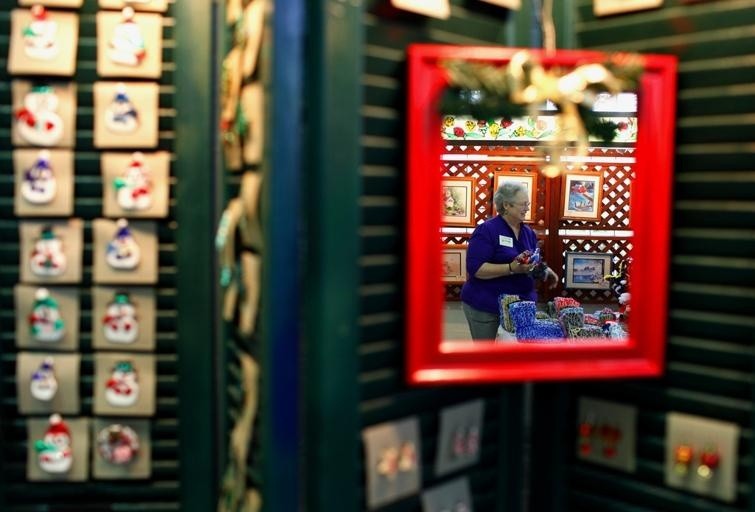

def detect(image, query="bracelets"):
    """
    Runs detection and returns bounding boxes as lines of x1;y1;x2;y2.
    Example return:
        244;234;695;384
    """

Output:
509;263;515;275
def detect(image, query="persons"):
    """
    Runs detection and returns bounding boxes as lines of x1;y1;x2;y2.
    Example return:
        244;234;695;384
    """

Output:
459;183;559;342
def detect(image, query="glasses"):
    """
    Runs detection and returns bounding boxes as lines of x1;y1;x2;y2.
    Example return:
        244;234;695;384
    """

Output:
507;201;530;207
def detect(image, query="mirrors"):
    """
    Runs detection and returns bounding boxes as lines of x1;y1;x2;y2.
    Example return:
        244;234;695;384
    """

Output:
405;44;678;386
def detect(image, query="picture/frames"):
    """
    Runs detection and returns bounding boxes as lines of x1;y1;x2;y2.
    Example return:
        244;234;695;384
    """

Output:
492;171;538;224
441;244;469;285
565;252;612;291
439;176;475;227
559;170;604;222
